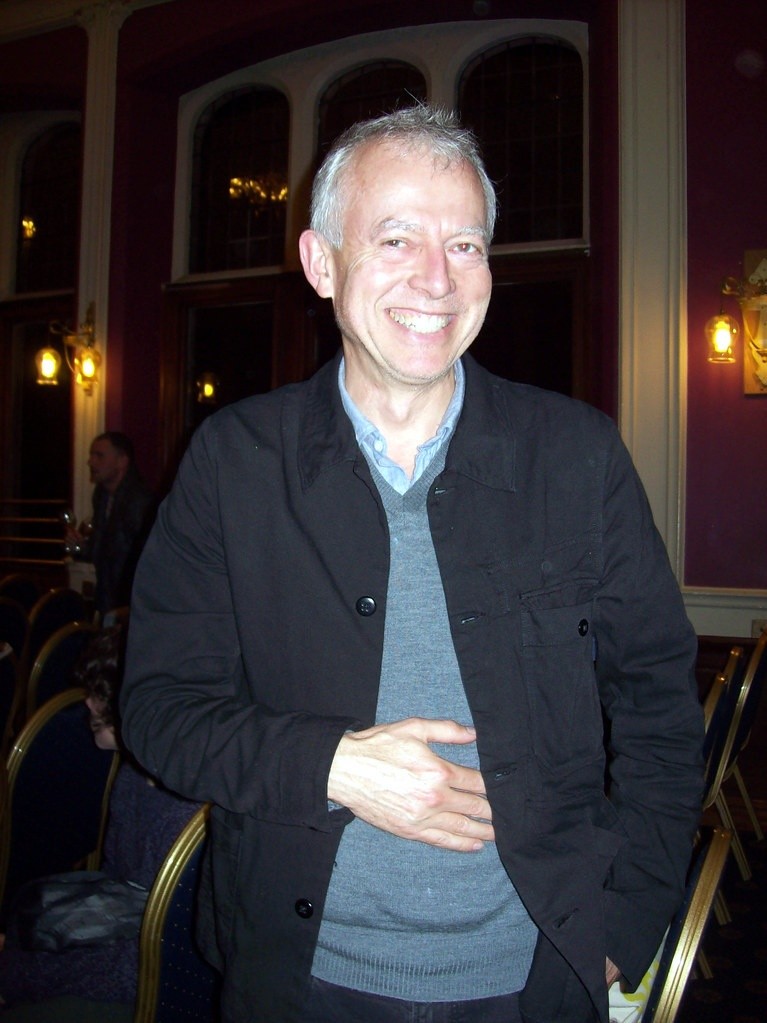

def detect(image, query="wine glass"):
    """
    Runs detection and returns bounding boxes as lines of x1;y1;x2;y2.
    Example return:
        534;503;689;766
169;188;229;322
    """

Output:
59;508;81;553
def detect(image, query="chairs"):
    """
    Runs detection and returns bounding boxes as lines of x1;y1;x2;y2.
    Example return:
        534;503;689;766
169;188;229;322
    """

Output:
0;571;767;1023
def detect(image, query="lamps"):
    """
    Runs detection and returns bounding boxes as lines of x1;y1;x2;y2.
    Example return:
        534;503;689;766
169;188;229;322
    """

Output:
32;303;101;387
704;250;767;395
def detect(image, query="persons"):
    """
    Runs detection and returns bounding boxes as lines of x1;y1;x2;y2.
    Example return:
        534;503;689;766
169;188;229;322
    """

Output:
0;618;225;1023
121;106;707;1023
69;431;156;608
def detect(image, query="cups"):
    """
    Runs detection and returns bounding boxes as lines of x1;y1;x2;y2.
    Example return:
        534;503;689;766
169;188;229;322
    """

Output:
78;517;94;539
